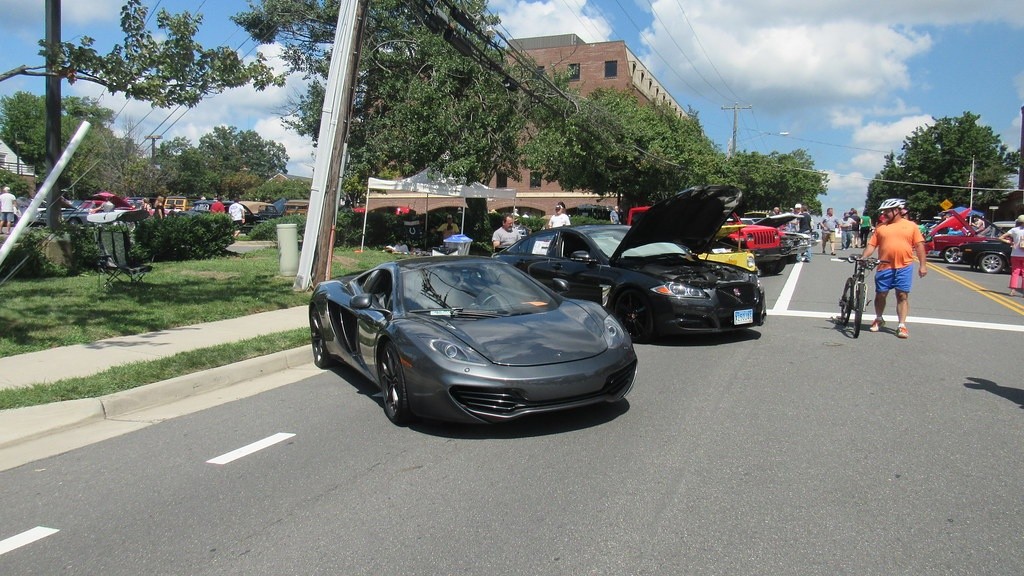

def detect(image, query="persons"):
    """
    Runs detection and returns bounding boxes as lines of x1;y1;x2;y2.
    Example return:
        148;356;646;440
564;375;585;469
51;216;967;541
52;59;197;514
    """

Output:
492;213;521;250
999;214;1024;297
860;198;927;338
212;197;225;213
0;187;17;235
973;216;985;232
437;214;459;238
610;206;621;224
101;197;114;212
820;207;871;255
549;204;570;229
140;195;165;218
408;205;416;217
229;198;245;236
773;204;812;263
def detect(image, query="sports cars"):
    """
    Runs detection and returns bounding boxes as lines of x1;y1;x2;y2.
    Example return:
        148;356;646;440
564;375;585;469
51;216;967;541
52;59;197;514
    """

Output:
308;254;638;426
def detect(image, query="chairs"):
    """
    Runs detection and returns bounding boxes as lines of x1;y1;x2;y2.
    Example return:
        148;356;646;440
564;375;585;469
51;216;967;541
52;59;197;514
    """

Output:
86;209;153;289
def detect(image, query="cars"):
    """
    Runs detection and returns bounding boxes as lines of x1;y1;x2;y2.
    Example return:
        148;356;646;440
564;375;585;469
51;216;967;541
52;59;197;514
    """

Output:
0;192;150;233
491;186;767;344
922;206;1014;274
727;212;812;263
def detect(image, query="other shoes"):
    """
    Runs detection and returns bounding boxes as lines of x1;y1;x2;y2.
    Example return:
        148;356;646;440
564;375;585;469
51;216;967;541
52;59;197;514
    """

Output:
831;252;836;255
802;257;810;263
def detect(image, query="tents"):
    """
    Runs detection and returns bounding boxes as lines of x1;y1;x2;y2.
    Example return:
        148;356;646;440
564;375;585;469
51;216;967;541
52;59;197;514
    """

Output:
361;151;517;252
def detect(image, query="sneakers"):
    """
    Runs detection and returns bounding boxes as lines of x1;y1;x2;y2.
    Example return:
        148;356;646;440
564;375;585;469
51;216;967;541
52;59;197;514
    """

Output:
869;319;885;332
896;326;908;338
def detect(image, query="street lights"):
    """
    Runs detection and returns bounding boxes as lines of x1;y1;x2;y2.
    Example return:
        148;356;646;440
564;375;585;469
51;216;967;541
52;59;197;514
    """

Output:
727;132;789;160
144;135;163;192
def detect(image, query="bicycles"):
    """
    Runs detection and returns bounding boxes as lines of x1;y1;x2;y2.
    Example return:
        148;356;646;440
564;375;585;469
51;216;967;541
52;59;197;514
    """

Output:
838;254;890;338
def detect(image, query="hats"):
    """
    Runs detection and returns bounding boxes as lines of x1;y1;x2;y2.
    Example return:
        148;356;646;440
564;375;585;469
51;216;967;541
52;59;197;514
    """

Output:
447;214;453;219
1017;215;1024;223
555;205;562;210
795;203;802;209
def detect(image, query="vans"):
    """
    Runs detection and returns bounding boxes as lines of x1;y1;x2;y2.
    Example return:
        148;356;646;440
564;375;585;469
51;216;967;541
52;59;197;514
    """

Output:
977;221;1016;238
127;196;283;235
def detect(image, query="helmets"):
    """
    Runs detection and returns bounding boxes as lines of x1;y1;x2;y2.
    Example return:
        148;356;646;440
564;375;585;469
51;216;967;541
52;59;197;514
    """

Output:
879;199;903;210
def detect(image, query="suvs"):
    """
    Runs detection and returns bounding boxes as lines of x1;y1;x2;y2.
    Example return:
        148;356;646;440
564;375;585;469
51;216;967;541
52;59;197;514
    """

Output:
627;206;794;275
